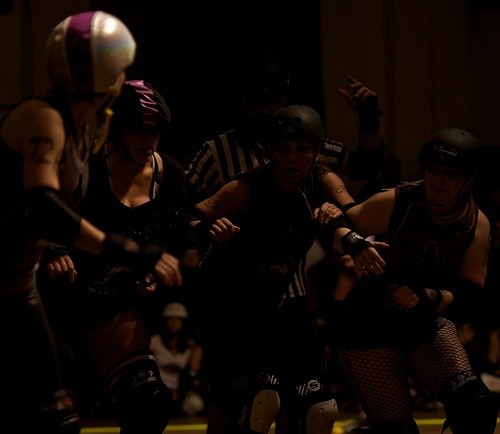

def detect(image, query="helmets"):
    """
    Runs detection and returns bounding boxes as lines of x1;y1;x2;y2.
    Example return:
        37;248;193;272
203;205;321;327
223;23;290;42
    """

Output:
161;301;189;319
264;105;326;144
110;79;171;129
416;124;481;169
40;11;137;95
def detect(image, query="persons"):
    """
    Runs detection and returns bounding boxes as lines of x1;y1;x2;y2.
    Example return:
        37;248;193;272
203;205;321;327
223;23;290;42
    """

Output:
316;128;491;434
1;10;385;434
